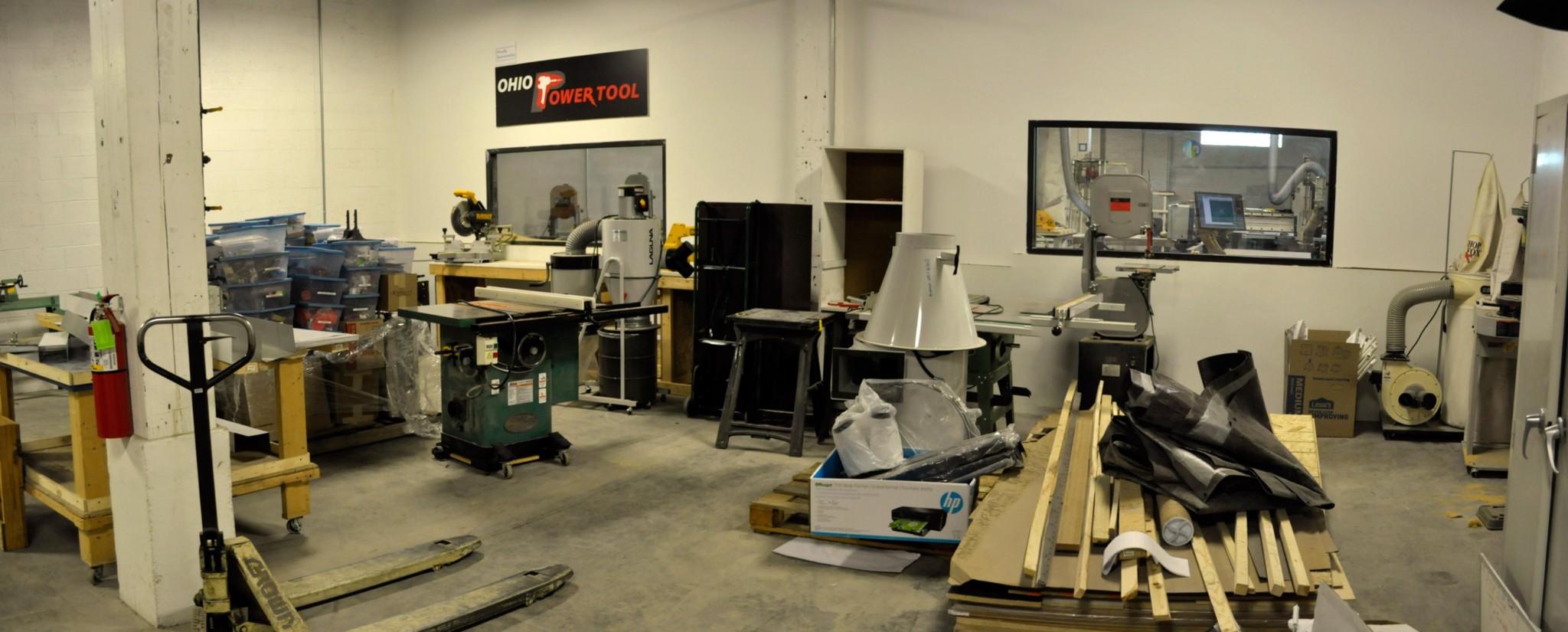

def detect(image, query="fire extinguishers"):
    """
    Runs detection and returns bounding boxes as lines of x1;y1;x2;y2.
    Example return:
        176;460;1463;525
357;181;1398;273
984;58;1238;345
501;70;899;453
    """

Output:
88;294;135;439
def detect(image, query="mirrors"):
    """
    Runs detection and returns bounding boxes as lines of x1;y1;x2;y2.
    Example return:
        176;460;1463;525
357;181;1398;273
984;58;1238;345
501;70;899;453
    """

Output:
1026;118;1339;267
486;138;667;246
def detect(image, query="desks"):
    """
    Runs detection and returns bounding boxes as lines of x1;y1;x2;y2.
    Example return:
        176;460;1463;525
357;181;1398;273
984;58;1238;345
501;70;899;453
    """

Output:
0;291;362;585
430;260;695;404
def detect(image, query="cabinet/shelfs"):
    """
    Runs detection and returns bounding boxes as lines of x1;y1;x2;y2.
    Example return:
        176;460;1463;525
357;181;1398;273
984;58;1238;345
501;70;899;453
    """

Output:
818;143;924;304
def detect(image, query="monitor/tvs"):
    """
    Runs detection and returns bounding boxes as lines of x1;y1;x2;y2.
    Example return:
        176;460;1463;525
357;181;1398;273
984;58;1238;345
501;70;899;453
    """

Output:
1193;190;1248;231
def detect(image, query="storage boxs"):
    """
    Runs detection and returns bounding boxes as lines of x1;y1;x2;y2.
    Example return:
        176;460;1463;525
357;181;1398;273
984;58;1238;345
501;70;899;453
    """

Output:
807;445;976;542
206;211;417;441
37;330;71;362
1284;325;1362;440
